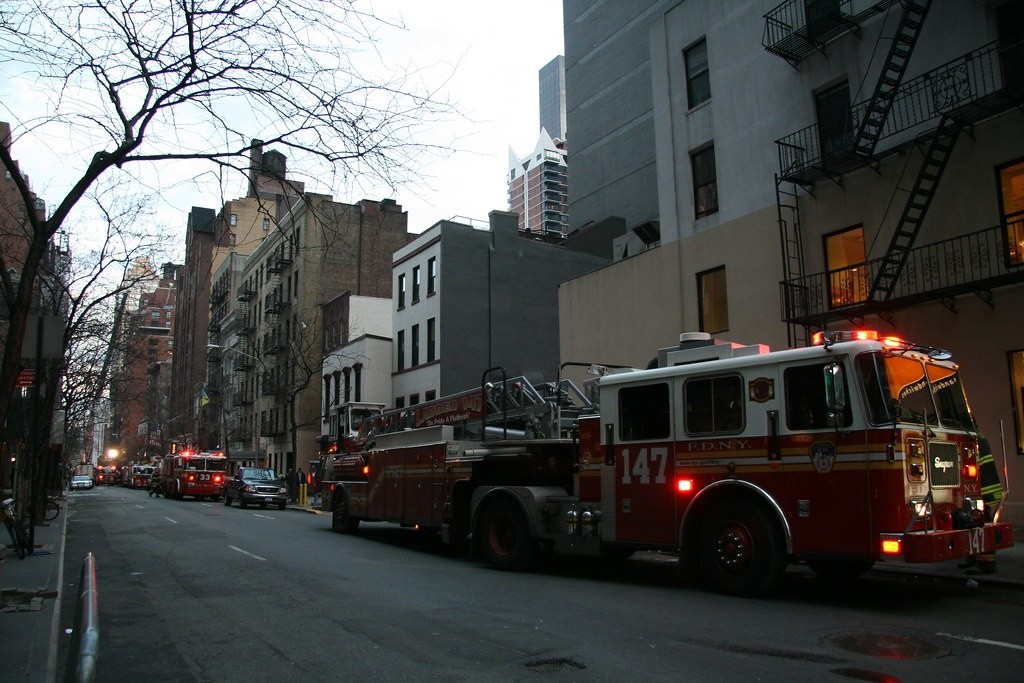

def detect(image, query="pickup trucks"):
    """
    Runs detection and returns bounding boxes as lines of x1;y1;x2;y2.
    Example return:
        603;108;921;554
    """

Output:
220;466;290;510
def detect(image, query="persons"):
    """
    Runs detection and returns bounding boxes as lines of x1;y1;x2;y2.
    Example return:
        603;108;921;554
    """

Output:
284;468;293;492
954;413;1003;572
147;471;161;498
296;467;306;502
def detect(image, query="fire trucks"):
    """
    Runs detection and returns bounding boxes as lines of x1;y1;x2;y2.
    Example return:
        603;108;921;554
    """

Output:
308;328;1016;600
93;451;231;502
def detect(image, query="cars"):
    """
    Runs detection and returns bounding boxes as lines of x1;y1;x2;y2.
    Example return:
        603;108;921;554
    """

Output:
71;475;93;491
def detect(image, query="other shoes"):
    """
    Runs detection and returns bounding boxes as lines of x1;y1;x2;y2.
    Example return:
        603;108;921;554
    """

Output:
964;560;998;575
958;562;975;569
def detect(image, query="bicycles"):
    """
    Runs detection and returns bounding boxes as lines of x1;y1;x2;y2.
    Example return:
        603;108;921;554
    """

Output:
0;487;61;561
